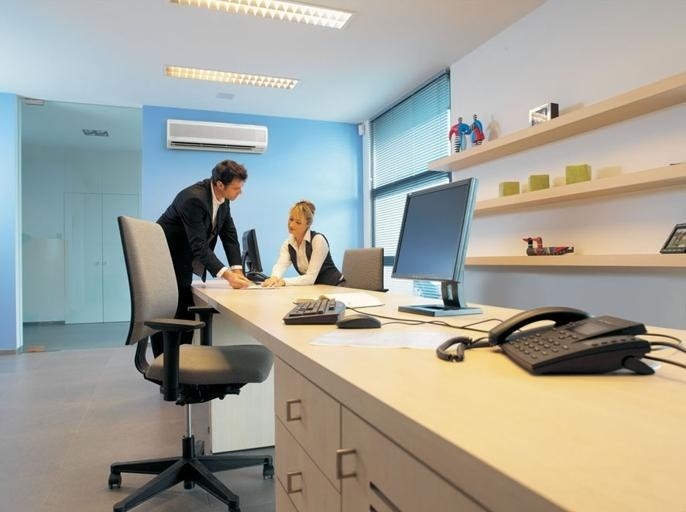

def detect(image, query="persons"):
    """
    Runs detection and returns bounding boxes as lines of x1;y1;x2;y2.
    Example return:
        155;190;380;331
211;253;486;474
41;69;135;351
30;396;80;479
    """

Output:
469;114;486;145
150;160;248;396
260;199;346;290
449;117;472;153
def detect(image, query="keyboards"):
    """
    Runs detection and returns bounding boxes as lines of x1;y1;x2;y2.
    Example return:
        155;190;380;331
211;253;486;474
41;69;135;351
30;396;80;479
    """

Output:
283;297;346;325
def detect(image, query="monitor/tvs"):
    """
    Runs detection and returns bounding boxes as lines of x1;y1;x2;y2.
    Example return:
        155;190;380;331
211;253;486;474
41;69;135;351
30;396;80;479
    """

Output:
391;176;480;315
240;228;264;285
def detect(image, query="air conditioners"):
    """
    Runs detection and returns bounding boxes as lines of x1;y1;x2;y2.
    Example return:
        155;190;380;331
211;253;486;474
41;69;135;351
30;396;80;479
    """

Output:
166;118;269;155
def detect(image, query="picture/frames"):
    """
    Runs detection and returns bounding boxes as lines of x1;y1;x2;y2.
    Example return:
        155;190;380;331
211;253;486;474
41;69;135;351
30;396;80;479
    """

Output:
660;223;686;254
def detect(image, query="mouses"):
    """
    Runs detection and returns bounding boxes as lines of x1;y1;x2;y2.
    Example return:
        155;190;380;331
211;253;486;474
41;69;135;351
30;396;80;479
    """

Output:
335;314;381;330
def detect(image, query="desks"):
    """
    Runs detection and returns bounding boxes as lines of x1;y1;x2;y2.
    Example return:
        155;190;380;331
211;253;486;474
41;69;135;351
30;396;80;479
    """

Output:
188;283;686;512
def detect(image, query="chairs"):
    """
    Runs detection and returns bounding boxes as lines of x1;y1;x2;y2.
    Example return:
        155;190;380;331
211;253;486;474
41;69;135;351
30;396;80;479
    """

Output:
107;212;275;512
340;248;384;292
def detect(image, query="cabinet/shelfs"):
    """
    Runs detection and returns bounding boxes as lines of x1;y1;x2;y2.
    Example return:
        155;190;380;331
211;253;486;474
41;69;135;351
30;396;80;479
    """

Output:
426;73;686;269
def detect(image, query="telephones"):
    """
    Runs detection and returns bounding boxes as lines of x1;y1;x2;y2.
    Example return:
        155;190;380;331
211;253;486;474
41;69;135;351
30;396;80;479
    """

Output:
489;307;655;375
